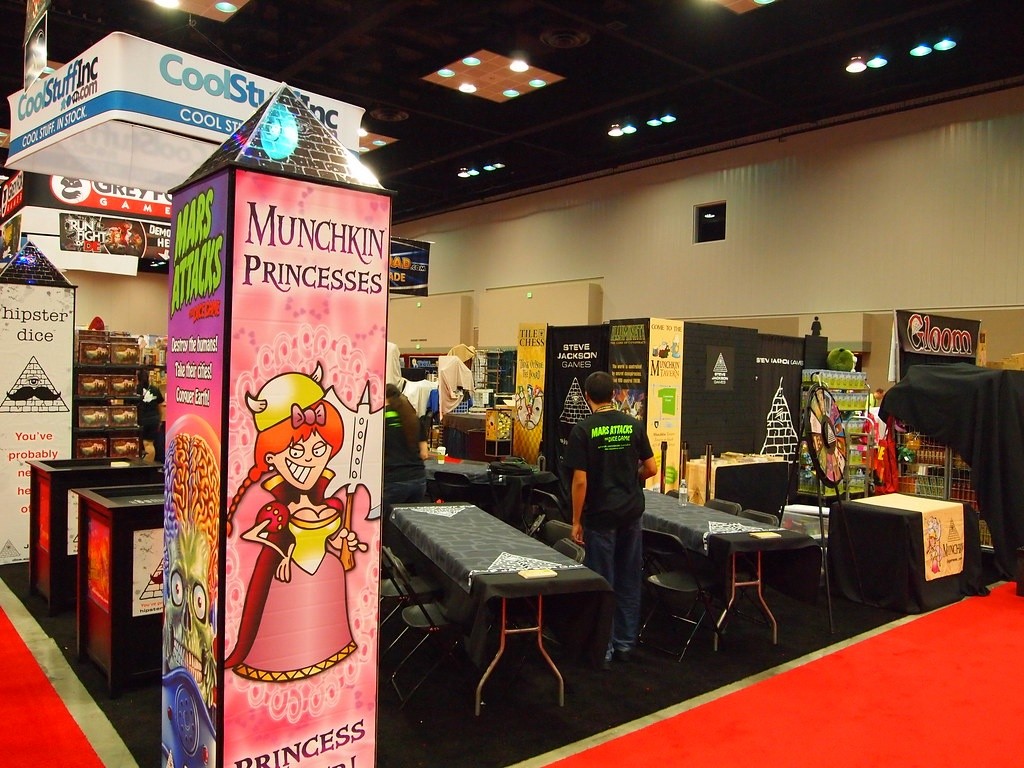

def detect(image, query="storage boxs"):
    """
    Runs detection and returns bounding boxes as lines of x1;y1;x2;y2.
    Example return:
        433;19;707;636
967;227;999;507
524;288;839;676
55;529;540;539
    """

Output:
75;328;139;460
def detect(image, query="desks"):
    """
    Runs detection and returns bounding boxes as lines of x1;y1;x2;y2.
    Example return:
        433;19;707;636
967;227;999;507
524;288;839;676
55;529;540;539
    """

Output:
387;501;614;715
684;454;790;526
424;452;566;537
641;488;822;652
443;411;504;464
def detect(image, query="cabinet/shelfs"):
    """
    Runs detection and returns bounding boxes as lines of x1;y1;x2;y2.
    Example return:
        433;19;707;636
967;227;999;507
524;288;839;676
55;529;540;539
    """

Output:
25;457;164;617
797;371;870;504
69;482;164;698
73;328;143;461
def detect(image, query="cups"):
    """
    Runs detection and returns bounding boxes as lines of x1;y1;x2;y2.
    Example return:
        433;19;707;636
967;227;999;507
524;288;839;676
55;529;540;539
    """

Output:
437;447;445;464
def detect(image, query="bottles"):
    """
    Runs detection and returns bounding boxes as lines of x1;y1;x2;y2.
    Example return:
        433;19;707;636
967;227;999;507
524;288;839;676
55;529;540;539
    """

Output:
678;479;687;506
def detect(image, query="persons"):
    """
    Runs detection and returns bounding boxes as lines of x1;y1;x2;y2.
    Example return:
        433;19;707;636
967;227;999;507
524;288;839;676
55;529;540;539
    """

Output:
137;369;164;463
564;371;658;661
383;384;429;558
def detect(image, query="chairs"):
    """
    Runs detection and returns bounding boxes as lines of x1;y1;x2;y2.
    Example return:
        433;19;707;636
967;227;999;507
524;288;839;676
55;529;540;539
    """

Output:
377;451;778;716
